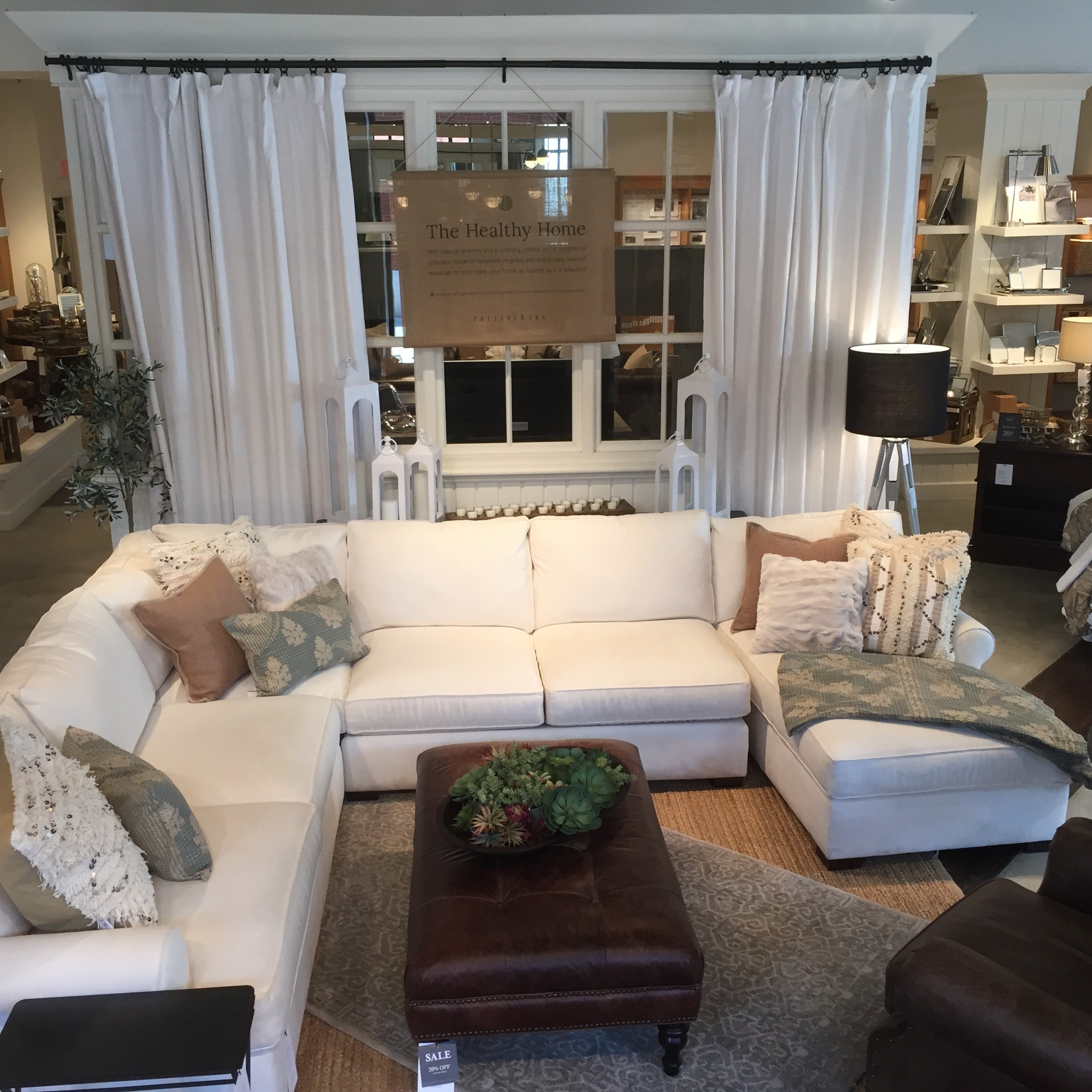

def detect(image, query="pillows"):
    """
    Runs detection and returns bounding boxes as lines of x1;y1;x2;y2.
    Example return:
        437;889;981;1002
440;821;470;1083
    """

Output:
624;343;655;369
1;507;973;934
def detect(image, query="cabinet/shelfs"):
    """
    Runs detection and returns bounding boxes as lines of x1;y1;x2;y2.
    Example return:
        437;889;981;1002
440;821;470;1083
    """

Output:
907;221;970;358
969;224;1090;376
0;228;89;532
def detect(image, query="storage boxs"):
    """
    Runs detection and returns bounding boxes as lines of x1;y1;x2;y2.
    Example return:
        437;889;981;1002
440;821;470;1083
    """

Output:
0;378;64;465
914;389;1032;445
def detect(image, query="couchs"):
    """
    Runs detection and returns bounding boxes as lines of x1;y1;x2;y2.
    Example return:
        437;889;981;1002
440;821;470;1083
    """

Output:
710;509;1072;870
364;323;683;439
850;815;1092;1092
0;511;753;1092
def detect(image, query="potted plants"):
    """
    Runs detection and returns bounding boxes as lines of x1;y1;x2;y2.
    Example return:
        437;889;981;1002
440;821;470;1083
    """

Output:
436;734;638;858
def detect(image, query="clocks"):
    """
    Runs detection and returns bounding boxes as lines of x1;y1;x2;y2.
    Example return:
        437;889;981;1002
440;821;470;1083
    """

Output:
923;108;939;160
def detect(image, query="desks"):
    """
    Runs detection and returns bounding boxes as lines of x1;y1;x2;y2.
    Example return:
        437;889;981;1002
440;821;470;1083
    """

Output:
401;727;707;1076
4;329;120;397
967;422;1092;573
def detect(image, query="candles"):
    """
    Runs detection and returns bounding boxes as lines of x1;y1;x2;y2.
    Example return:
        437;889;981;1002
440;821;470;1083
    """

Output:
339;454;706;521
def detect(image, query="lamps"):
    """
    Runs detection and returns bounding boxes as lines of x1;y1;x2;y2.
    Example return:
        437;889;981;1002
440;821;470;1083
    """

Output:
526;123;549;168
1059;316;1092;444
845;343;951;536
52;251;72;287
999;145;1059;226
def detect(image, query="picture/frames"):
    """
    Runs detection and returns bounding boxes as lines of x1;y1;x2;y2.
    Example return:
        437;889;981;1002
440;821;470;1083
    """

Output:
911;248;939;345
925;154;1049;227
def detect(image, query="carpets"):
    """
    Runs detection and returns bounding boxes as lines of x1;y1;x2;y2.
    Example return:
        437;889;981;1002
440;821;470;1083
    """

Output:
306;789;927;1091
